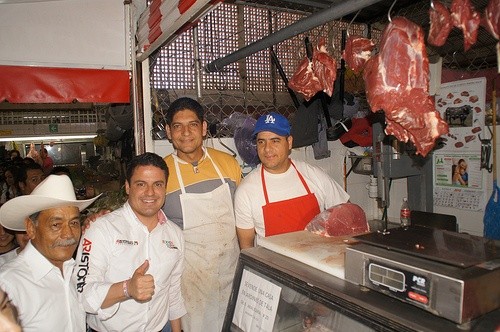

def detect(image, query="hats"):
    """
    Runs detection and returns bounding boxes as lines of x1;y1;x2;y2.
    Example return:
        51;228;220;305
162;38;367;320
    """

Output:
252;112;290;139
0;174;103;231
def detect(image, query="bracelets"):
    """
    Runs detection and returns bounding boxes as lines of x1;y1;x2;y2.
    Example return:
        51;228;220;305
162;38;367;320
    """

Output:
123;280;130;298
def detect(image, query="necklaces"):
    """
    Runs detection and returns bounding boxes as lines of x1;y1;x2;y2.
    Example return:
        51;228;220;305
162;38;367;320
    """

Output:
177;155;204;174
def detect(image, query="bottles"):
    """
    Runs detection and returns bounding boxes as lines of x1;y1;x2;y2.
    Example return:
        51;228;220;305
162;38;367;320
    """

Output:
401;197;412;226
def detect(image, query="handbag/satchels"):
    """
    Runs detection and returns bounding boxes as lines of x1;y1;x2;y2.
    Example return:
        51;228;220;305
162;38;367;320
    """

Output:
483;186;500;239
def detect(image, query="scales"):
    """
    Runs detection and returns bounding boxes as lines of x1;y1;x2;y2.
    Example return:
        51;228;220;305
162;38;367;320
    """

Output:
343;225;500;329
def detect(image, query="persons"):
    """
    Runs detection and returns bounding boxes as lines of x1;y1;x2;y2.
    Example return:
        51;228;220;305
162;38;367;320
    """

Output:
158;97;243;332
234;111;351;251
70;152;187;332
0;174;102;332
0;287;23;332
452;159;468;186
0;143;57;269
